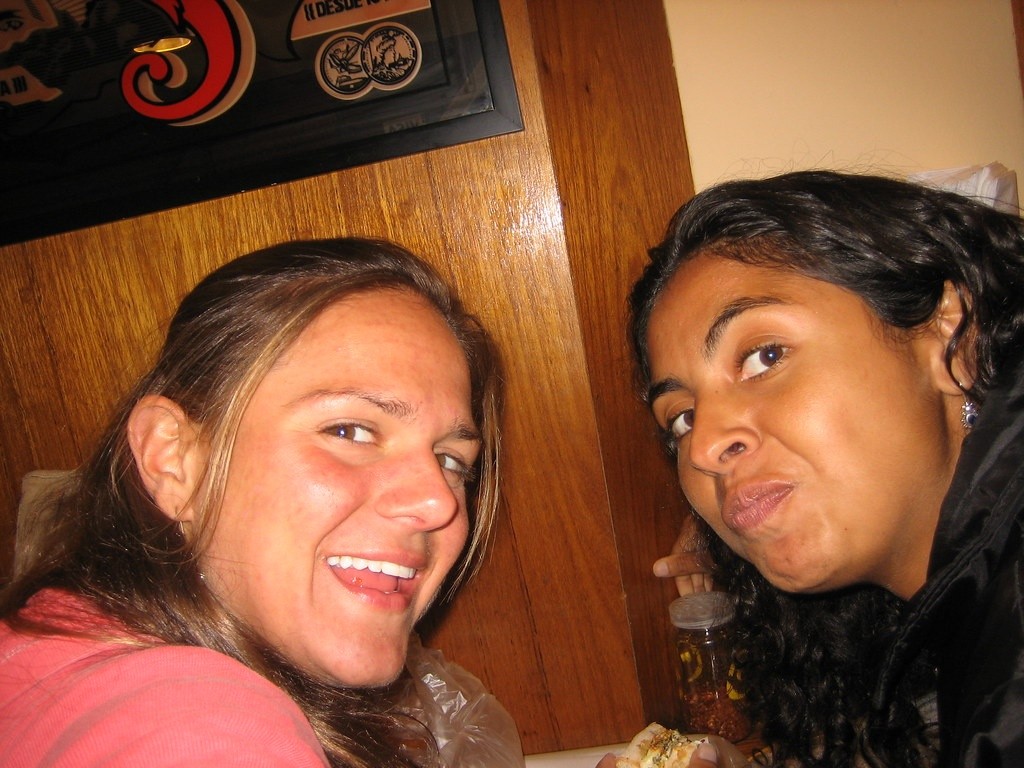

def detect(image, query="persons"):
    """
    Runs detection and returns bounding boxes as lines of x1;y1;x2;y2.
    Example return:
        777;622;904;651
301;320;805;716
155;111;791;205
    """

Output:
594;173;1024;768
0;235;503;768
